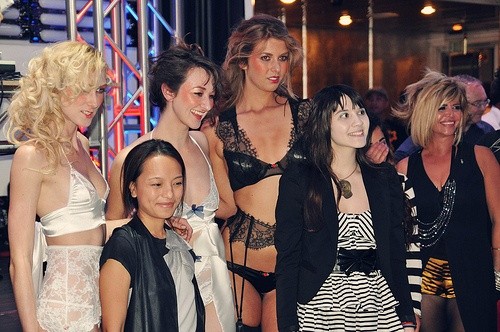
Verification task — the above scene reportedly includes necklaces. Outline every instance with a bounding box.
[337,162,359,199]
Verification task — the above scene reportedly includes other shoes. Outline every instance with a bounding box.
[30,31,41,43]
[17,29,29,40]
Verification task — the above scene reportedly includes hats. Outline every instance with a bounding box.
[363,86,390,99]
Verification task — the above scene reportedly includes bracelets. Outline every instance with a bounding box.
[401,322,416,328]
[493,248,500,291]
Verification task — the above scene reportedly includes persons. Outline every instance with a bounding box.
[363,66,500,165]
[1,40,110,332]
[365,112,422,332]
[273,84,417,332]
[396,72,500,332]
[199,14,314,332]
[99,139,206,332]
[105,35,237,332]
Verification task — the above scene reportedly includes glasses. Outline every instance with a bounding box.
[467,98,490,108]
